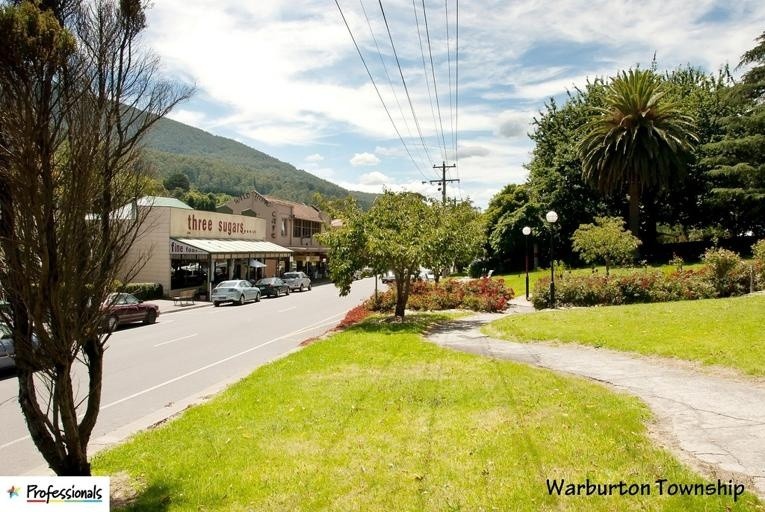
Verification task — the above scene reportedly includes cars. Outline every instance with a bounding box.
[252,276,290,298]
[210,277,262,307]
[0,291,162,380]
[170,263,227,281]
[380,271,395,284]
[424,268,435,280]
[281,271,313,293]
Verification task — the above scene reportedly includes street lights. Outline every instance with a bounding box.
[544,209,560,308]
[521,226,533,301]
